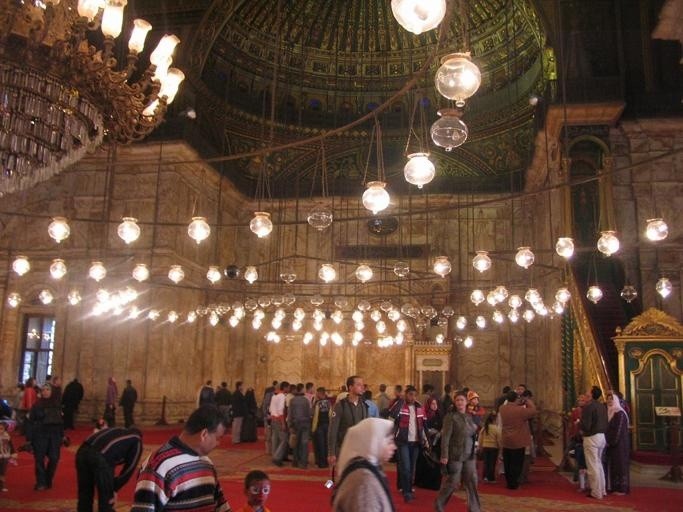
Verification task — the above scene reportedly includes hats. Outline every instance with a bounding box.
[467,391,479,401]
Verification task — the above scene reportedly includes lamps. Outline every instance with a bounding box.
[249,211,273,239]
[207,265,222,284]
[587,286,603,305]
[244,266,258,284]
[187,217,212,244]
[117,216,142,245]
[362,180,390,215]
[306,203,333,232]
[597,231,619,255]
[434,52,482,107]
[391,0,447,35]
[356,262,372,282]
[655,275,672,298]
[473,250,492,273]
[49,259,67,278]
[1,1,191,168]
[280,270,296,283]
[645,218,669,242]
[89,262,107,280]
[619,280,638,302]
[430,109,469,150]
[47,216,71,244]
[168,265,185,283]
[556,237,574,259]
[394,261,410,278]
[12,255,30,275]
[403,154,436,188]
[319,264,336,283]
[0,280,569,346]
[433,256,451,278]
[133,264,148,282]
[515,247,535,269]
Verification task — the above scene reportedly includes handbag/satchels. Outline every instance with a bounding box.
[289,434,297,449]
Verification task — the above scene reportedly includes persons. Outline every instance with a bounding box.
[119,379,137,428]
[93,415,109,434]
[131,405,231,511]
[261,376,543,511]
[1,375,84,492]
[566,385,631,499]
[241,469,271,511]
[75,425,144,511]
[103,376,118,426]
[198,379,258,445]
